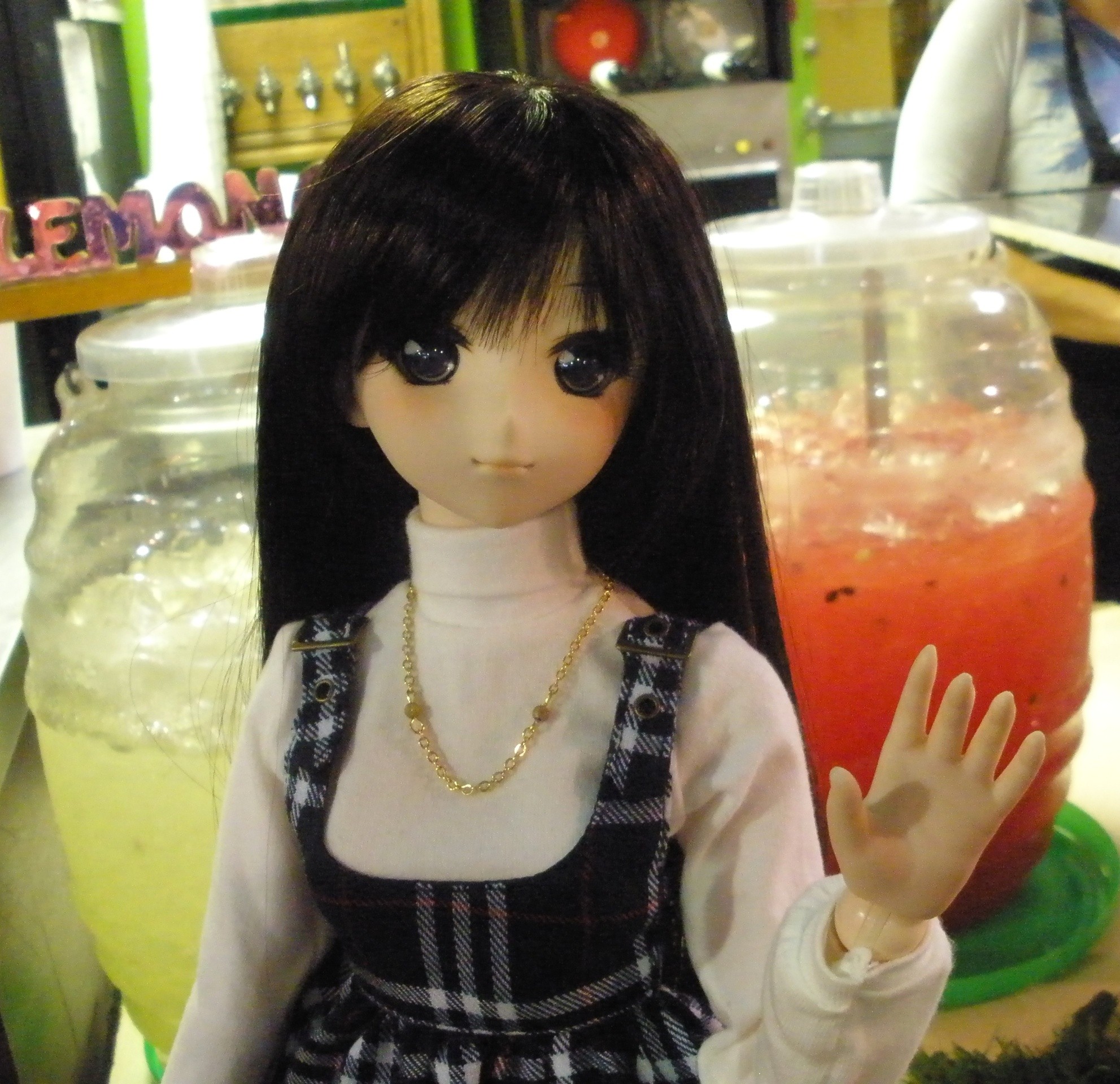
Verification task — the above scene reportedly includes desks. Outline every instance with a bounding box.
[865,187,1120,595]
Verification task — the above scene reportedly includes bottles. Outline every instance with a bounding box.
[548,0,649,94]
[660,0,769,86]
[23,232,265,1063]
[702,159,1096,934]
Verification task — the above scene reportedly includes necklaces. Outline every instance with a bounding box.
[400,564,611,796]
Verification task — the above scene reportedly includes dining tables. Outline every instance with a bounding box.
[108,596,1120,1084]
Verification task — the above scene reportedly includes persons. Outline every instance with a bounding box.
[885,0,1118,602]
[153,68,1052,1084]
